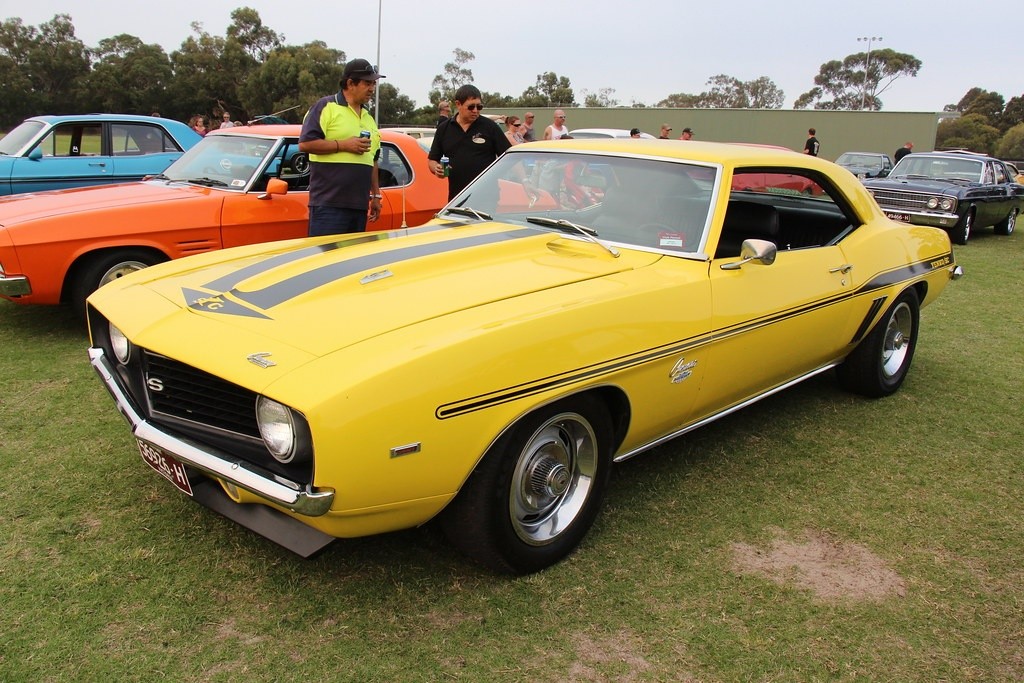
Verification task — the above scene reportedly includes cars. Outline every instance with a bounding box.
[10,125,561,316]
[84,138,951,575]
[0,113,288,203]
[826,147,1024,244]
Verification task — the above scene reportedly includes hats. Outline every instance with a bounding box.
[683,127,696,135]
[342,59,386,81]
[661,123,672,131]
[630,129,640,134]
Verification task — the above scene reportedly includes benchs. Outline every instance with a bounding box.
[663,196,848,248]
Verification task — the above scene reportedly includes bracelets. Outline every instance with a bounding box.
[334,140,339,154]
[372,193,383,200]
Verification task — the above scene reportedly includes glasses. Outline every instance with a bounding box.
[529,115,534,118]
[512,123,521,127]
[554,116,565,118]
[468,104,483,110]
[224,117,229,118]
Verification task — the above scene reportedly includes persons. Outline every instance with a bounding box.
[804,128,820,157]
[152,112,245,137]
[893,141,913,166]
[428,84,593,218]
[299,58,387,237]
[628,123,695,140]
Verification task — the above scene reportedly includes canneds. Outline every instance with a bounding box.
[440,157,450,177]
[360,130,370,149]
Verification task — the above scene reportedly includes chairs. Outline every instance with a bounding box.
[720,201,780,257]
[592,187,661,245]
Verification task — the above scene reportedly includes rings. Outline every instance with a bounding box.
[436,171,438,174]
